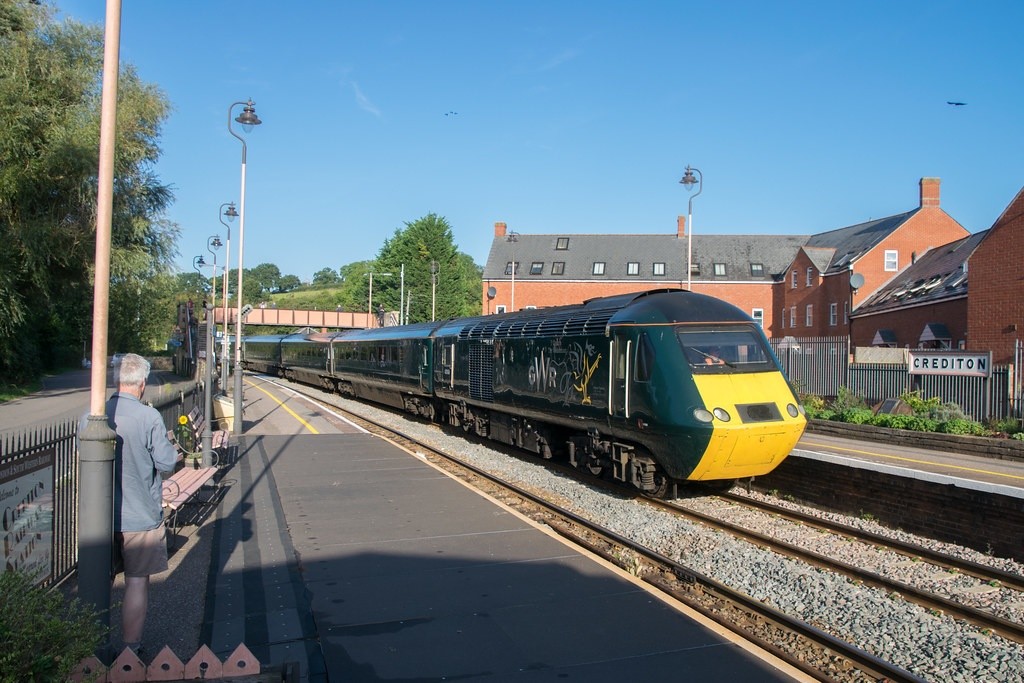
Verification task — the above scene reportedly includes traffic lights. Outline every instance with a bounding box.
[241,304,253,317]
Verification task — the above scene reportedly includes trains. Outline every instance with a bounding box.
[214,288,807,501]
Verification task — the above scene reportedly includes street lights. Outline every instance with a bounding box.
[369,272,393,314]
[220,202,240,396]
[506,230,521,312]
[193,254,206,351]
[228,97,263,435]
[679,164,704,292]
[175,326,182,375]
[208,234,223,356]
[428,258,440,322]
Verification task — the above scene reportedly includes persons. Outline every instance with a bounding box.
[704,346,726,364]
[77,352,178,665]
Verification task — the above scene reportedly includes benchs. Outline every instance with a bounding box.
[153,430,218,551]
[187,406,229,467]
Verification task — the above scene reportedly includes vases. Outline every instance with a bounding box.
[176,456,204,471]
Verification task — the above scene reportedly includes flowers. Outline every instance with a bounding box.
[177,416,191,444]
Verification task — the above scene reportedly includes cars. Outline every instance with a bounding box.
[110,354,124,368]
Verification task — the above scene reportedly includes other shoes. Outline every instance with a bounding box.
[122,643,147,662]
